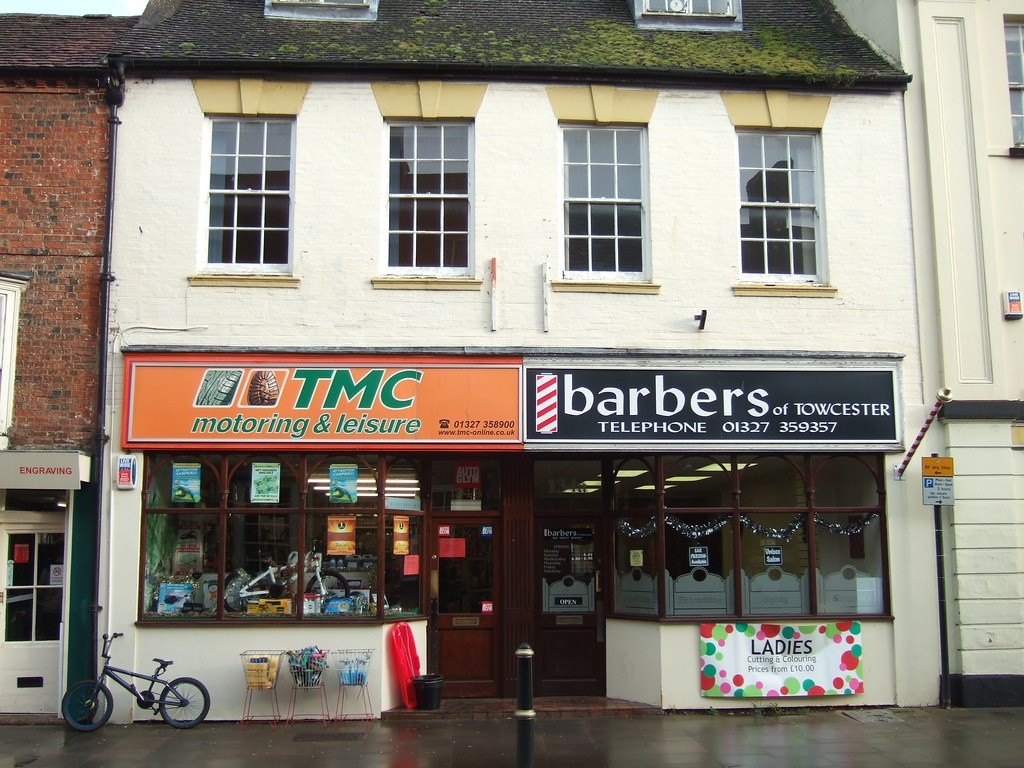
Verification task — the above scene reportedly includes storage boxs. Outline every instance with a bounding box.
[259,599,295,615]
[157,583,194,615]
[370,594,390,610]
[349,589,370,611]
[326,589,345,599]
[247,604,258,614]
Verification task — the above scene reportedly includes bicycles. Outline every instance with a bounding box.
[61,633,212,732]
[224,538,351,613]
